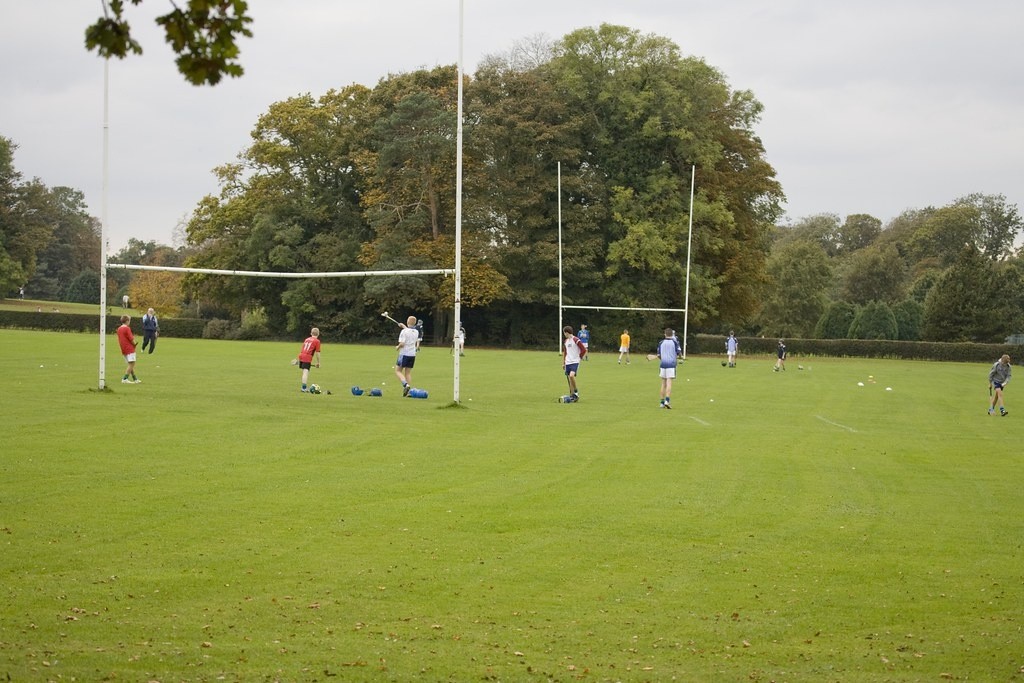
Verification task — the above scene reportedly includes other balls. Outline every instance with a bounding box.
[384,312,389,315]
[469,398,473,402]
[382,382,386,386]
[687,378,690,382]
[710,399,714,402]
[392,367,395,369]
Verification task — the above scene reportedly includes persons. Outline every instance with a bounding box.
[725,331,738,368]
[452,322,467,356]
[986,355,1012,417]
[617,329,631,365]
[52,308,59,313]
[122,294,129,309]
[773,338,787,373]
[117,315,142,384]
[141,307,158,355]
[298,328,321,392]
[657,328,682,410]
[395,315,424,397]
[37,306,42,313]
[562,324,589,402]
[18,284,25,300]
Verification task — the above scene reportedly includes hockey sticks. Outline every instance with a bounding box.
[290,359,321,369]
[989,387,998,417]
[381,311,404,328]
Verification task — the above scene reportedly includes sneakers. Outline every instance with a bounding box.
[134,380,142,383]
[122,379,133,384]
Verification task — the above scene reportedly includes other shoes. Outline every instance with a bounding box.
[988,409,993,414]
[402,384,410,397]
[660,404,664,408]
[574,392,579,401]
[664,401,671,408]
[301,388,309,392]
[1001,410,1008,416]
[141,348,144,354]
